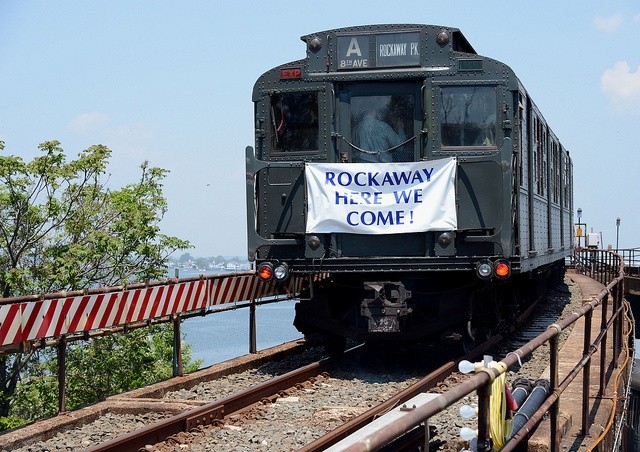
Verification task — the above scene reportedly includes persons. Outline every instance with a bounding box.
[352,101,406,164]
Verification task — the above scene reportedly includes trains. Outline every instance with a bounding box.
[243,23,575,375]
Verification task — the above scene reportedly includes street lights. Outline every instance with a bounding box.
[576,208,583,276]
[616,217,621,253]
